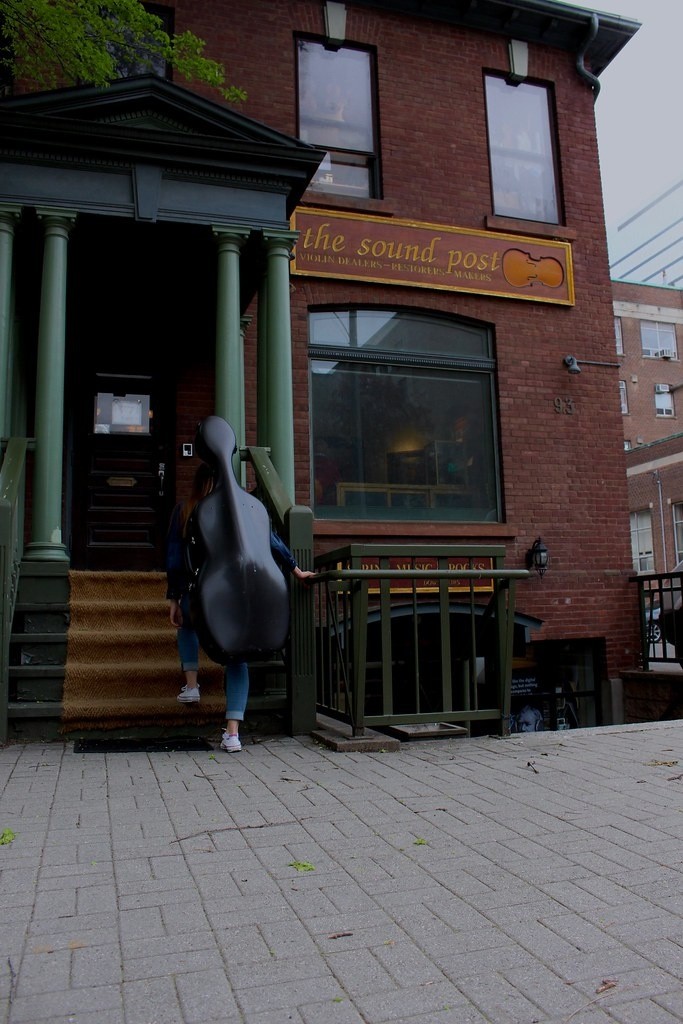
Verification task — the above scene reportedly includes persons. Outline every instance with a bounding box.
[167,463,315,753]
[515,704,543,734]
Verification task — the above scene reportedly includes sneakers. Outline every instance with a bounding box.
[220,726,242,753]
[177,683,202,703]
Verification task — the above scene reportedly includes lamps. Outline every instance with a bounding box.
[525,535,549,579]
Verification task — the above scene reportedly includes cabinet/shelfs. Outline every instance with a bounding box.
[335,440,482,508]
